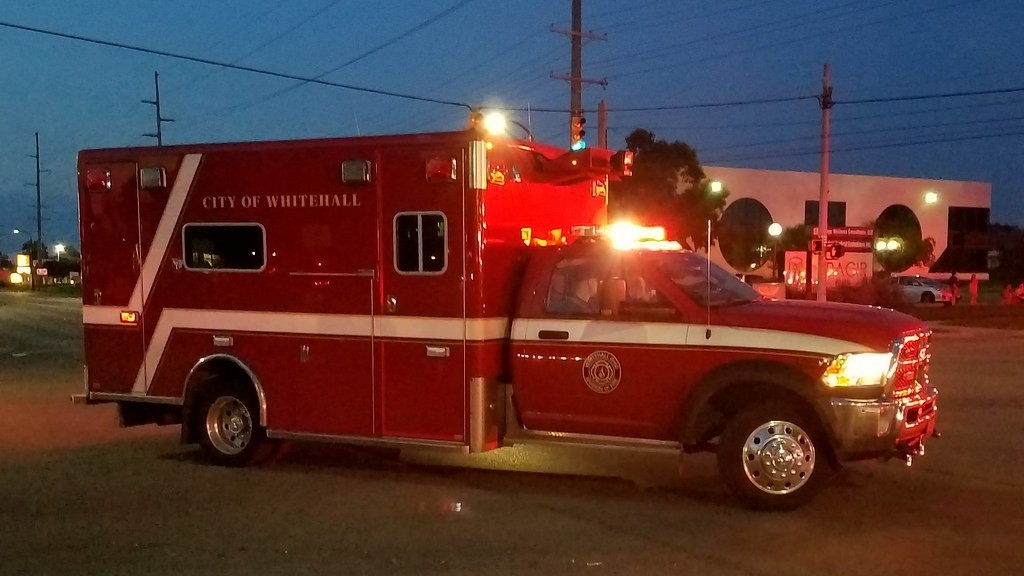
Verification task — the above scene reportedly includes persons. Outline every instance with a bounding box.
[949,270,959,306]
[70,279,74,285]
[546,260,601,315]
[970,274,979,305]
[1001,283,1024,305]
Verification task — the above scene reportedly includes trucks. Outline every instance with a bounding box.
[68,131,939,508]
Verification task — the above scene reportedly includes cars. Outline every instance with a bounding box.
[889,274,953,306]
[734,273,764,287]
[70,276,80,285]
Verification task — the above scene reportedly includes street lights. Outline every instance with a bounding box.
[13,230,32,259]
[877,239,898,271]
[56,243,65,261]
[767,222,781,281]
